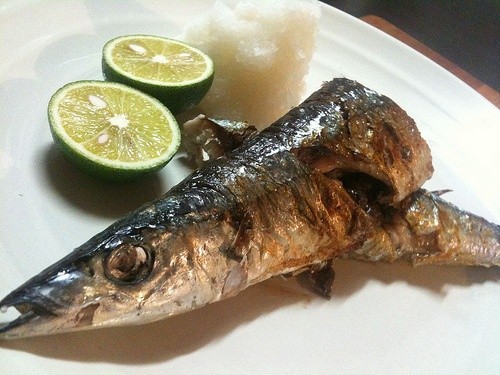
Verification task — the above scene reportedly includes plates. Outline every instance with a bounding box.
[0,0,499,374]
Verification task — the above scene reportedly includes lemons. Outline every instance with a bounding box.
[48,34,216,183]
